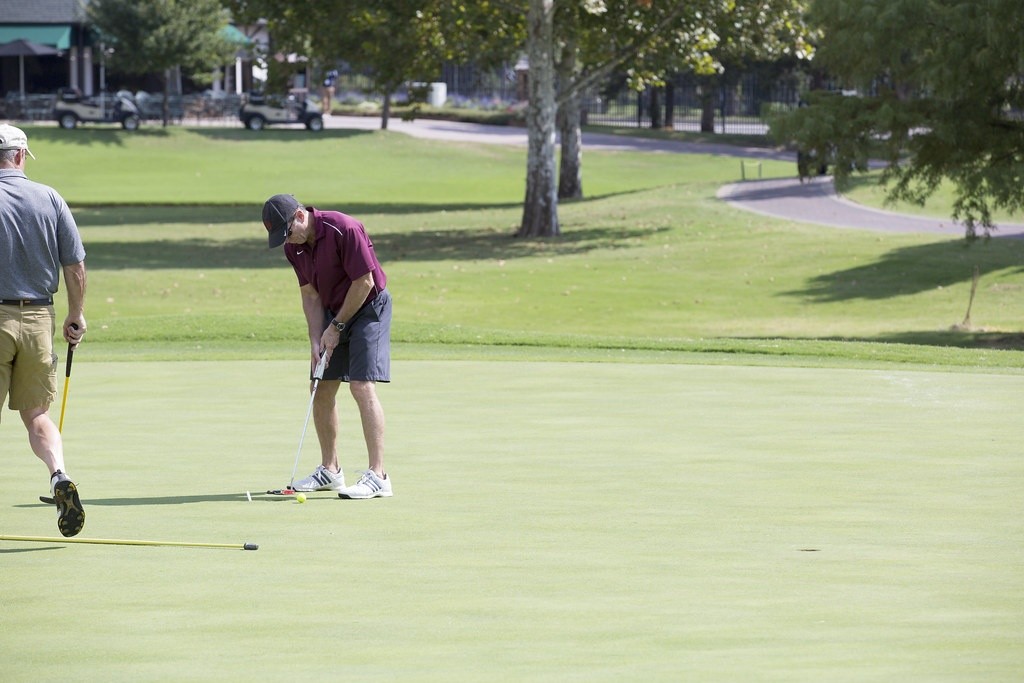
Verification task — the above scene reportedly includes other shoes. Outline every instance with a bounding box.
[52,474,85,538]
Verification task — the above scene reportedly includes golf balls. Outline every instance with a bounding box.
[297,494,307,502]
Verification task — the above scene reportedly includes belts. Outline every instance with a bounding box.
[0,298,53,305]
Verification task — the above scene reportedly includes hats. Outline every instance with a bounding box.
[0,124,36,160]
[262,194,298,249]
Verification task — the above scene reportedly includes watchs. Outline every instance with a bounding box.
[332,319,345,331]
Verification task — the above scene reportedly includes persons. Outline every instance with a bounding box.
[0,124,86,536]
[117,86,149,125]
[263,195,393,499]
[321,70,336,120]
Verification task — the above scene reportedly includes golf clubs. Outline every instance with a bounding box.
[3,534,261,551]
[266,348,327,496]
[39,322,80,504]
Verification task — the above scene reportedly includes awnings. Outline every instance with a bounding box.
[0,26,71,49]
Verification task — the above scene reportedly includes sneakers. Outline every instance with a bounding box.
[338,465,394,499]
[287,464,345,491]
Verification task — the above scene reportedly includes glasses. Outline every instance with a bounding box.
[288,213,296,236]
